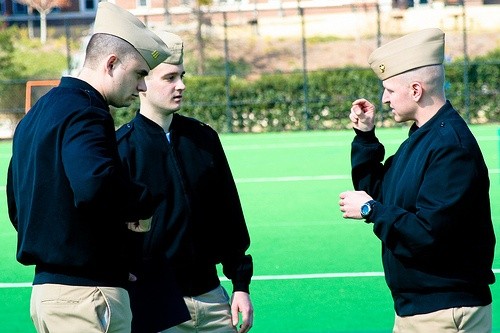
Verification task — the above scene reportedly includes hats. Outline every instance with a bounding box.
[150,25,184,65]
[368,28,446,81]
[93,1,172,70]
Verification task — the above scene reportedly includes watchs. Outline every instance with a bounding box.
[360,200,377,219]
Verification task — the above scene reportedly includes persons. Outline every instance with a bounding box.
[115,31,253,333]
[7,3,171,332]
[339,28,496,333]
